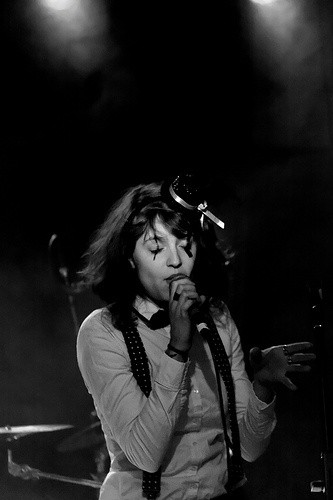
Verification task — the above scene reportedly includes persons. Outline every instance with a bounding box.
[73,173,316,500]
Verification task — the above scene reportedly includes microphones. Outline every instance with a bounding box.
[170,274,211,341]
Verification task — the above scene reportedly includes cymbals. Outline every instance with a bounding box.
[0,423,75,444]
[54,421,105,453]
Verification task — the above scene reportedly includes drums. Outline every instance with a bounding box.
[228,448,248,492]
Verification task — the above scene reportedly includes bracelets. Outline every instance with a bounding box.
[166,343,189,359]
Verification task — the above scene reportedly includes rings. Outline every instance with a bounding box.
[282,344,290,355]
[287,354,293,365]
